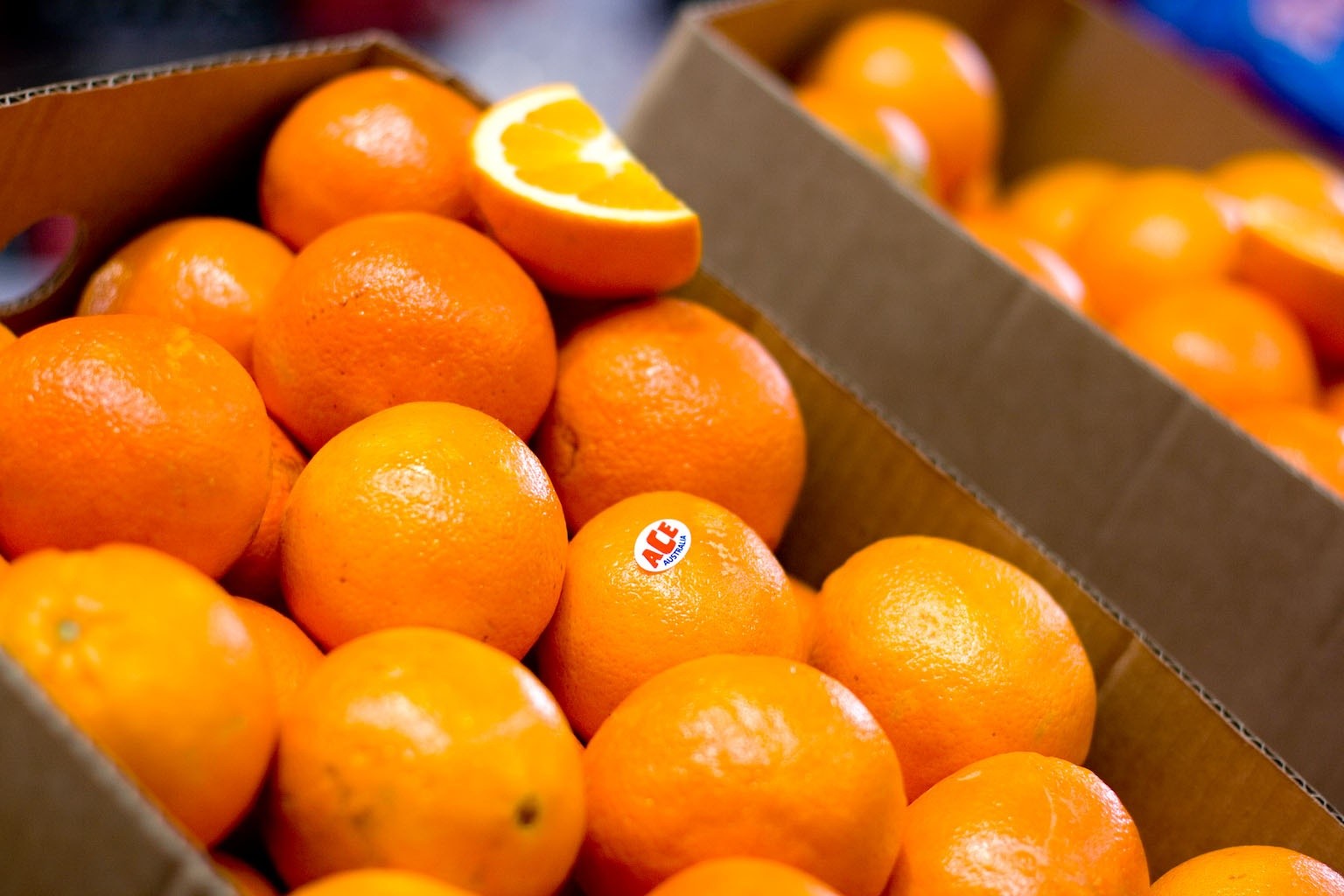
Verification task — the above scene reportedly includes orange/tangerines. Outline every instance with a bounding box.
[773,11,1344,519]
[0,63,1344,896]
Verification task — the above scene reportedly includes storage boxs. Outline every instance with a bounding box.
[1,2,1342,892]
